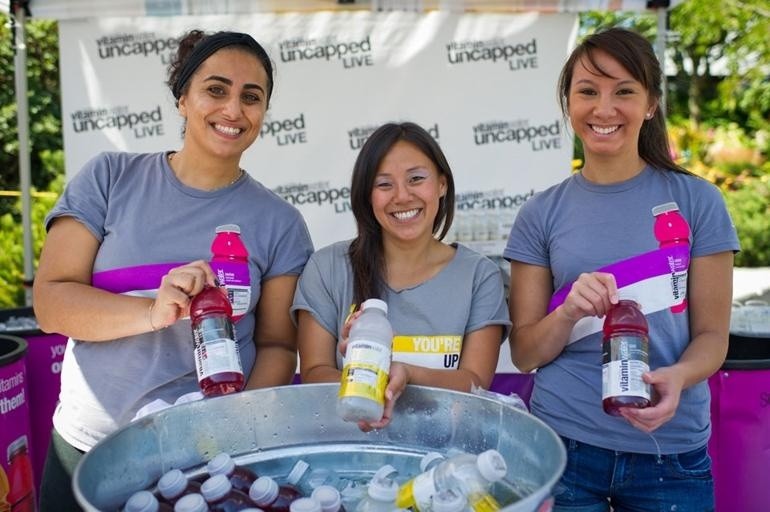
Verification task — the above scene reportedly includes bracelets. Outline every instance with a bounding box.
[149,300,168,333]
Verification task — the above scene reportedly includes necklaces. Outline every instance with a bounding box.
[168,152,243,185]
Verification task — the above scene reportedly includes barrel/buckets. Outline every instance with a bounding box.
[72,381,568,512]
[0,336,39,511]
[0,307,69,486]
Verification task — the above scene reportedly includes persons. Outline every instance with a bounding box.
[31,28,313,511]
[501,25,742,512]
[289,120,515,432]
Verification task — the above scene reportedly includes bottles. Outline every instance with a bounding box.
[188,272,250,398]
[120,448,511,511]
[209,223,255,322]
[8,433,37,511]
[652,200,695,313]
[336,292,398,427]
[601,290,654,417]
